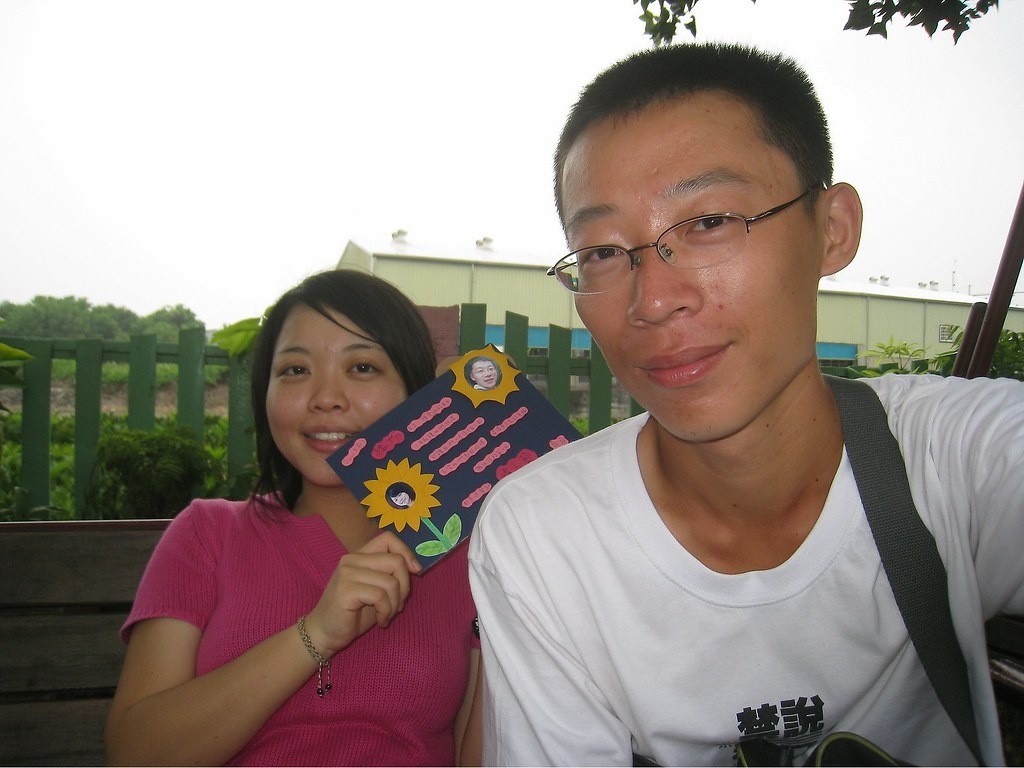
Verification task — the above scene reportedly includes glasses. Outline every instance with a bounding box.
[545,180,829,295]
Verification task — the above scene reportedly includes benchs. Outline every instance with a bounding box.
[0,531,170,768]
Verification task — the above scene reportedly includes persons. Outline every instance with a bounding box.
[101,267,486,768]
[466,42,1024,767]
[386,481,417,512]
[469,357,499,391]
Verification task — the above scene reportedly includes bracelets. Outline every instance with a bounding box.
[296,616,332,697]
